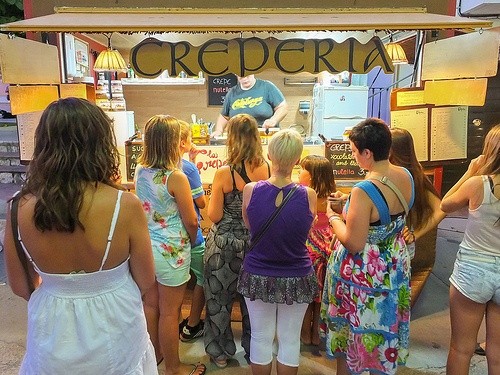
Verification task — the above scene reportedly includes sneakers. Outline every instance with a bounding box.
[180,319,206,342]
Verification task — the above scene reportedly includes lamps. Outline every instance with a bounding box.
[93,33,128,72]
[385,31,409,65]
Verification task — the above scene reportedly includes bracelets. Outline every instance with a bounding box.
[411,233,416,243]
[328,215,341,223]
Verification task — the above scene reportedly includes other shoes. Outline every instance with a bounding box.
[474,342,487,356]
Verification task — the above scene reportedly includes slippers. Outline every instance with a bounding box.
[178,361,207,375]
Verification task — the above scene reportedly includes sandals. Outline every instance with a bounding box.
[210,354,228,368]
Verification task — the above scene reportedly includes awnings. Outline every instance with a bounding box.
[0,6,493,33]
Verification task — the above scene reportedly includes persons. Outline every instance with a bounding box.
[133,114,206,375]
[202,113,273,369]
[4,97,160,375]
[388,128,448,261]
[210,73,288,138]
[178,120,206,342]
[319,117,415,375]
[441,122,500,375]
[297,155,337,347]
[235,128,319,375]
